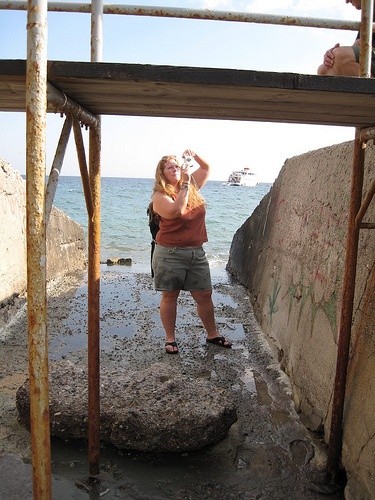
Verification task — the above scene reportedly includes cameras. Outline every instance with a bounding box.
[185,156,194,167]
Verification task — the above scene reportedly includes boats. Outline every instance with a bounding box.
[227,167,259,191]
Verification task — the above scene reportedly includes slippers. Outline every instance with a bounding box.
[165,341,178,354]
[206,335,232,348]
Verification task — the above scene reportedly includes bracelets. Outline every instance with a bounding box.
[181,181,188,191]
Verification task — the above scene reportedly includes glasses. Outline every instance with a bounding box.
[162,155,177,160]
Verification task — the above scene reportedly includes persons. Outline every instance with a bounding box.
[150,149,232,354]
[317,0,375,76]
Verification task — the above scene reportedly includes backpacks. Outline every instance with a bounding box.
[147,202,160,278]
